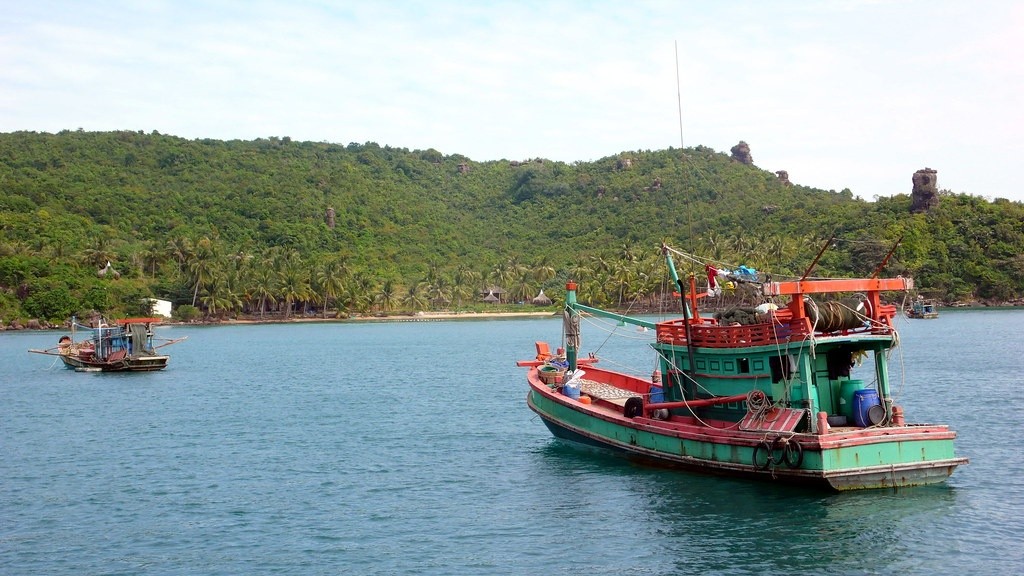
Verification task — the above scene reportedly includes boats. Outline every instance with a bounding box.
[515,232,970,493]
[905,292,940,319]
[28,313,188,372]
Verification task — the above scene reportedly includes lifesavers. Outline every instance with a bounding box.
[623,396,644,419]
[752,435,806,473]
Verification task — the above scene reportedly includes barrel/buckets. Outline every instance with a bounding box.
[853,389,880,427]
[542,367,559,372]
[538,365,565,384]
[840,380,864,423]
